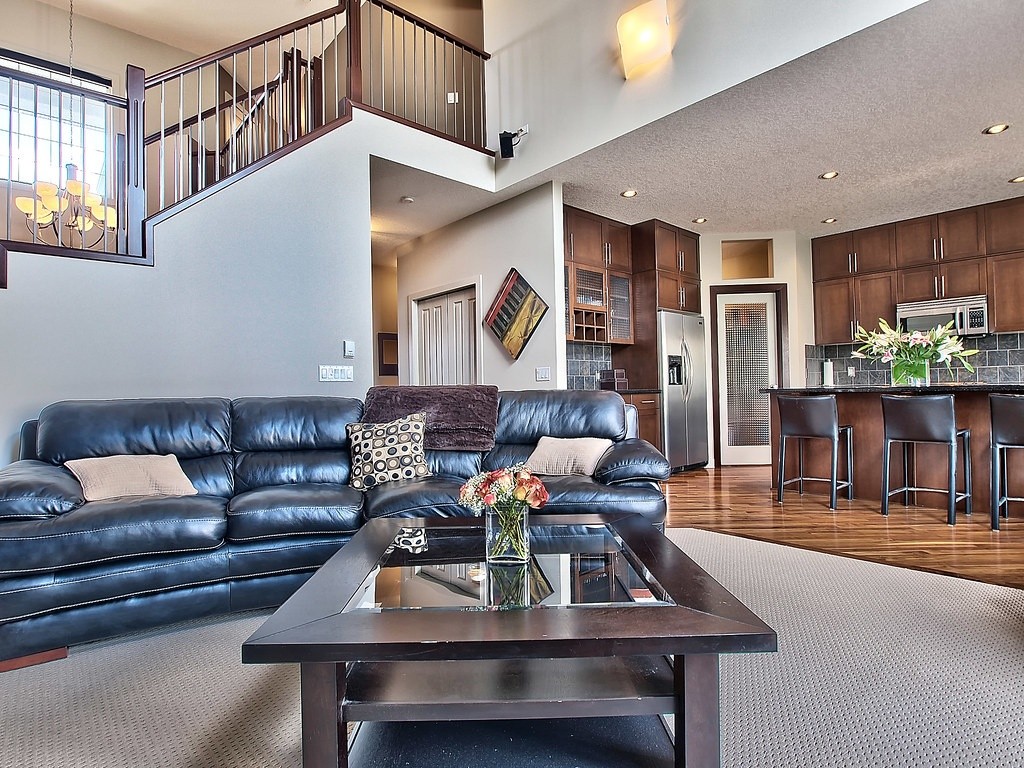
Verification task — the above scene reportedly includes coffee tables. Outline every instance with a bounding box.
[241,513,779,767]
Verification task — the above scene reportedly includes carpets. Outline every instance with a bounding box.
[0,526,1023,768]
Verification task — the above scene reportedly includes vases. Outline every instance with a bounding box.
[486,560,531,607]
[891,359,930,387]
[486,501,531,559]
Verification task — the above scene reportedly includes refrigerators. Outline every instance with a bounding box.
[657,309,707,472]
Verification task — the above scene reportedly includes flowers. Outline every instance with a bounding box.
[849,318,979,383]
[458,462,549,559]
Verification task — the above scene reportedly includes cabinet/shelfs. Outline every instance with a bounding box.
[618,394,661,454]
[811,195,1024,347]
[563,204,701,345]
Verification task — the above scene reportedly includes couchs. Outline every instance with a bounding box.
[0,389,671,673]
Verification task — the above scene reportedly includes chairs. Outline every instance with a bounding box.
[777,394,854,511]
[879,394,972,524]
[989,394,1023,532]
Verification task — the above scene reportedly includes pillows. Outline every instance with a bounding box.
[64,453,198,501]
[526,435,615,476]
[345,411,433,491]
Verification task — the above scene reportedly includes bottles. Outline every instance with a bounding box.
[824,359,834,385]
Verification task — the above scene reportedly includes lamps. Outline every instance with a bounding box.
[15,0,119,250]
[616,0,671,83]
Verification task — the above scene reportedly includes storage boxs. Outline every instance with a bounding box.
[599,369,628,391]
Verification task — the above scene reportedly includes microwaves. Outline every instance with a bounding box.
[895,295,988,339]
